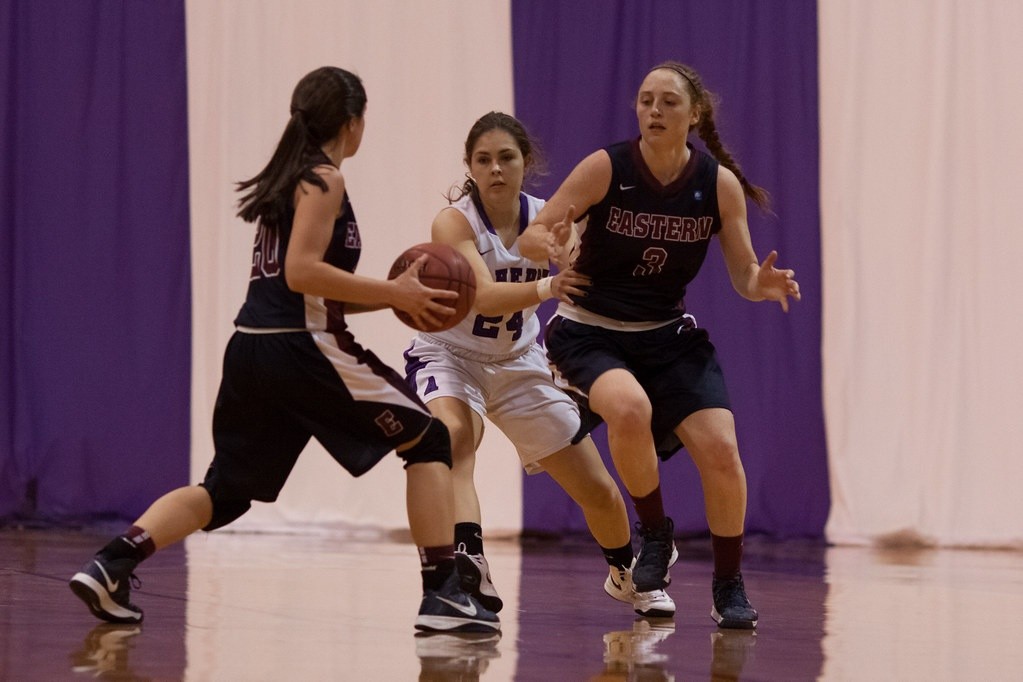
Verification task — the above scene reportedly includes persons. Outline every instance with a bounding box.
[402,111,676,617]
[517,61,801,629]
[68,66,500,633]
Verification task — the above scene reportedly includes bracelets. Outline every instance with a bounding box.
[536,276,554,302]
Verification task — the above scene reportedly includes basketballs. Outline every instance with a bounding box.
[387,242,477,333]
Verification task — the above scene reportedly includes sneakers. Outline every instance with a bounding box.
[454,543,503,614]
[414,574,501,633]
[711,574,759,630]
[632,518,679,593]
[69,554,144,623]
[603,557,677,617]
